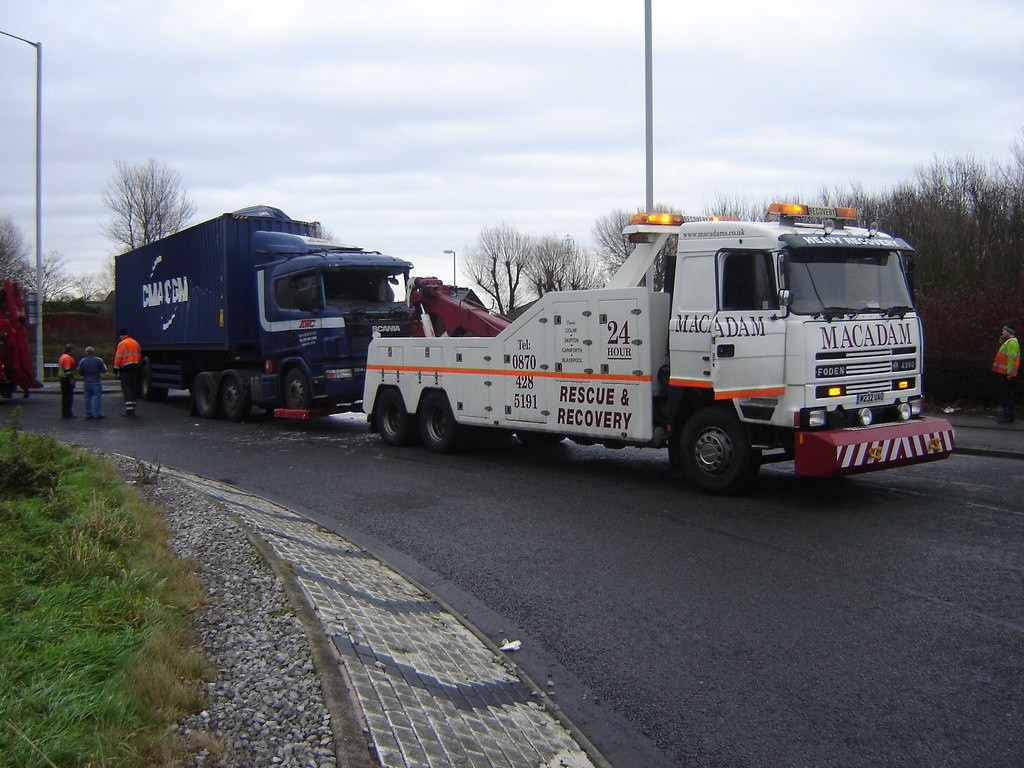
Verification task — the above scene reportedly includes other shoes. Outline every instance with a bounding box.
[121,412,133,417]
[94,415,105,419]
[87,415,96,419]
[68,415,77,419]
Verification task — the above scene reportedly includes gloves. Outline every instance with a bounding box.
[69,379,77,390]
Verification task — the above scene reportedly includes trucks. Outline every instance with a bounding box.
[0,277,45,398]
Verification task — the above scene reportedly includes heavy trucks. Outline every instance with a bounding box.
[115,203,419,423]
[361,203,957,499]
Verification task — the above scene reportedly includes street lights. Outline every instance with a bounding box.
[444,250,456,288]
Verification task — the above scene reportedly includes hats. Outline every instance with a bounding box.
[119,328,128,335]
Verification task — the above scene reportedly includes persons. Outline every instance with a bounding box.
[76,345,108,419]
[992,324,1020,424]
[114,329,141,419]
[58,344,77,419]
